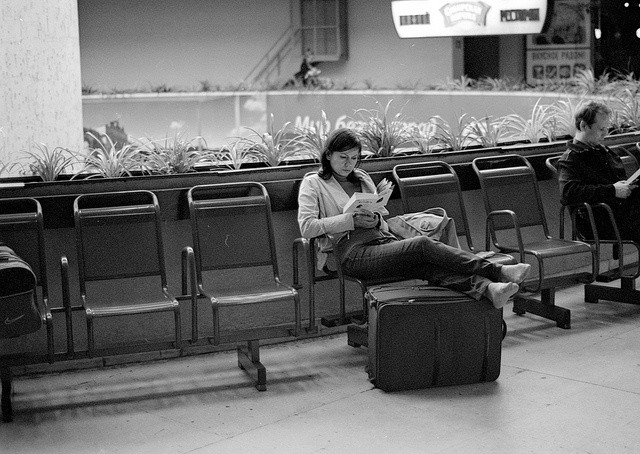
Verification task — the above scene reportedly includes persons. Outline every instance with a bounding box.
[296,125,532,311]
[553,100,640,249]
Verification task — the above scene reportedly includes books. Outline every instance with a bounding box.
[342,177,395,216]
[623,164,640,189]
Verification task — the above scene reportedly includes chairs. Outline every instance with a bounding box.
[72,189,183,355]
[392,161,518,279]
[0,197,55,424]
[186,180,301,394]
[542,151,640,303]
[472,152,601,329]
[292,176,405,326]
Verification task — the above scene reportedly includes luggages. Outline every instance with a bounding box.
[363,283,507,392]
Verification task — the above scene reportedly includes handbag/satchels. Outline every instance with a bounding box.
[0,242,43,339]
[386,207,462,251]
